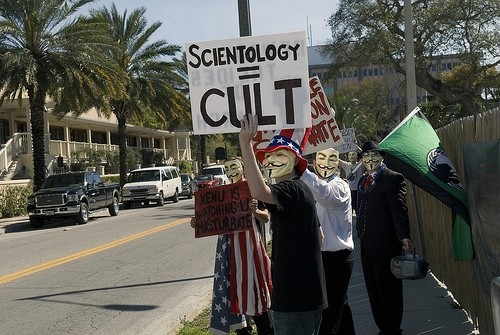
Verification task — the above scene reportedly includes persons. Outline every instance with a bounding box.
[355,140,412,335]
[339,150,366,238]
[238,113,329,335]
[299,148,356,335]
[190,156,275,335]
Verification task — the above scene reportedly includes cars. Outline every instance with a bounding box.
[193,174,220,191]
[179,174,198,200]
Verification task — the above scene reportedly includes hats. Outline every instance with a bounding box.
[255,135,308,178]
[357,140,385,159]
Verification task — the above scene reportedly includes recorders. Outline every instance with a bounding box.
[391,247,428,280]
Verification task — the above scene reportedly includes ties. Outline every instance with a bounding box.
[364,175,373,190]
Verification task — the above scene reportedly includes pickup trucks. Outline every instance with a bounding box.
[26,170,122,226]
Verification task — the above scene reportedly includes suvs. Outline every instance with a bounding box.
[202,164,231,185]
[121,166,182,208]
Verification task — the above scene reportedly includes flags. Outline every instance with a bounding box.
[377,108,474,262]
